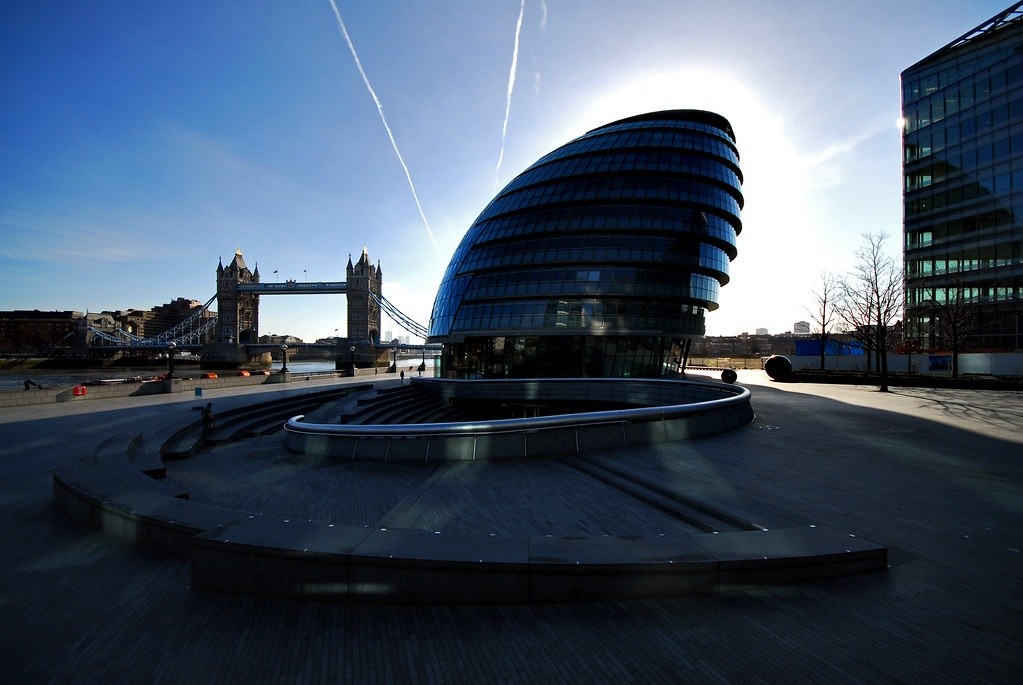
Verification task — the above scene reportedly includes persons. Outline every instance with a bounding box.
[201,402,215,434]
[400,370,405,384]
